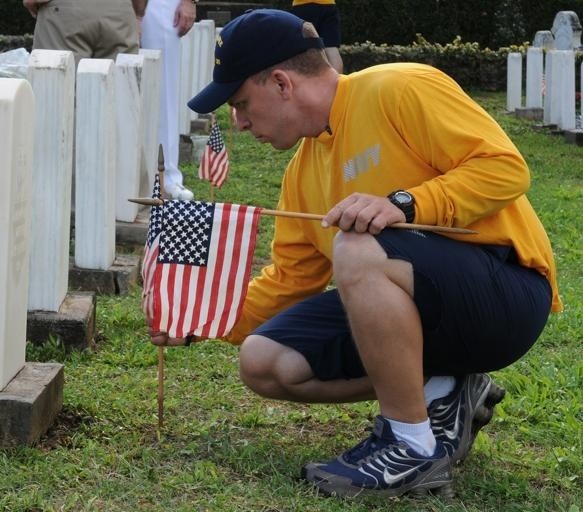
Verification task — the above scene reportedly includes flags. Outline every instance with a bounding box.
[151,196,261,341]
[199,122,231,187]
[140,173,162,320]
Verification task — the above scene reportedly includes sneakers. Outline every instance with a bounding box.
[301,373,504,498]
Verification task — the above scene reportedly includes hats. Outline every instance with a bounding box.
[187,8,325,114]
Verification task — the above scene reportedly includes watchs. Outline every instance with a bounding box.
[384,189,415,224]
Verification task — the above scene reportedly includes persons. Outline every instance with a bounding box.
[22,0,147,60]
[185,7,562,501]
[291,0,345,68]
[140,1,200,202]
